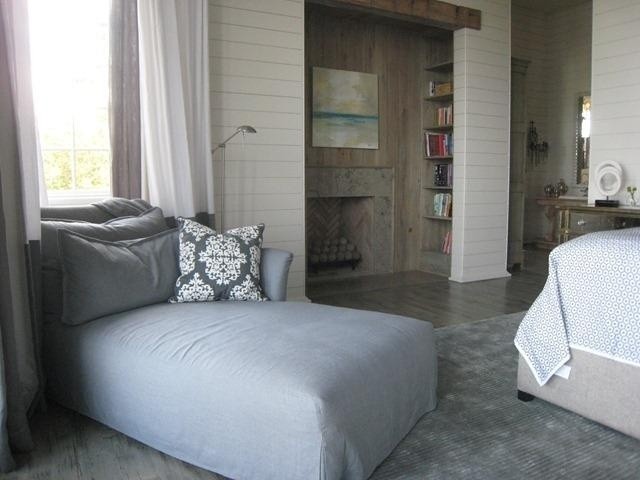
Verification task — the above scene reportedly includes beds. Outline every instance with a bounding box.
[516,224,639,441]
[32,198,437,475]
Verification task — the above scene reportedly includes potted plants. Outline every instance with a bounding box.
[627,186,639,207]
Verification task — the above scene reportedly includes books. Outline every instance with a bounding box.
[424,103,453,256]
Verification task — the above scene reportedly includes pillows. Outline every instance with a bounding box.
[36,207,271,327]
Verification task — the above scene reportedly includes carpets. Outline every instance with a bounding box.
[0,306,639,475]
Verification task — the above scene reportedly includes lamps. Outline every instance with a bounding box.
[210,124,256,162]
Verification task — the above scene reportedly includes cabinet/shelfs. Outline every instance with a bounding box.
[422,32,454,277]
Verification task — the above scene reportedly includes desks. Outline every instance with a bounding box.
[557,205,640,247]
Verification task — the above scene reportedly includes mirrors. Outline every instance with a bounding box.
[576,92,589,187]
